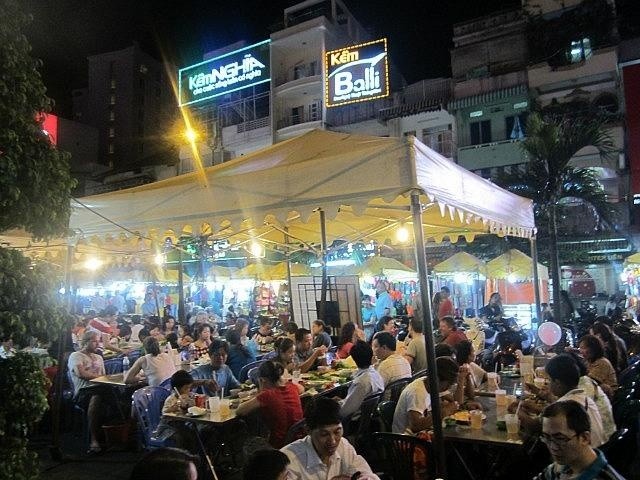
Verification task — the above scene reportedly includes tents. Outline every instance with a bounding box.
[150,268,191,285]
[52,126,548,480]
[230,263,266,278]
[260,260,309,281]
[360,256,419,285]
[205,266,235,278]
[433,252,488,302]
[623,251,640,268]
[487,247,549,305]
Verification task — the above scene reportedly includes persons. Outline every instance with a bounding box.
[390,357,467,480]
[518,353,607,451]
[482,331,524,371]
[335,321,367,358]
[178,324,195,350]
[593,316,626,353]
[311,319,333,349]
[255,337,296,376]
[67,331,112,454]
[372,332,412,400]
[22,336,47,353]
[439,317,471,353]
[566,348,618,440]
[234,317,249,346]
[549,302,554,317]
[532,400,627,480]
[226,330,256,381]
[389,283,403,316]
[117,337,176,444]
[0,338,14,359]
[284,322,298,338]
[603,296,621,318]
[333,342,385,419]
[86,306,131,356]
[191,313,217,335]
[457,339,488,388]
[251,321,276,344]
[376,281,393,321]
[375,316,397,336]
[588,323,628,374]
[156,369,199,480]
[577,336,618,397]
[189,340,244,395]
[437,286,453,325]
[227,305,237,321]
[282,396,382,479]
[141,292,155,317]
[479,293,506,331]
[138,326,150,343]
[542,302,549,318]
[188,323,212,361]
[560,290,574,315]
[131,315,144,340]
[163,316,175,337]
[167,333,179,360]
[434,342,465,393]
[294,328,327,373]
[244,446,292,480]
[404,318,427,368]
[149,323,160,337]
[236,359,305,449]
[361,295,376,342]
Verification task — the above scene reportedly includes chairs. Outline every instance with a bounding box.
[377,396,399,432]
[286,419,310,443]
[131,385,181,445]
[387,371,415,397]
[234,359,267,379]
[263,350,278,359]
[412,368,428,382]
[369,432,438,480]
[614,353,640,449]
[103,358,127,374]
[119,349,142,360]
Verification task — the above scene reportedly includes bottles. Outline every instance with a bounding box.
[123,357,130,377]
[189,343,195,361]
[195,394,205,407]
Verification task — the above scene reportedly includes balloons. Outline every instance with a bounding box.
[537,322,562,350]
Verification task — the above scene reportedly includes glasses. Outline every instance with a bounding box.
[538,432,579,445]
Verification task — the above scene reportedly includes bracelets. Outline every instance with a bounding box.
[466,371,472,378]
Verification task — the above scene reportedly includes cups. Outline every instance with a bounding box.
[495,389,505,404]
[219,400,231,416]
[470,409,481,430]
[487,372,498,388]
[504,414,519,434]
[293,371,300,381]
[534,378,545,388]
[205,397,218,413]
